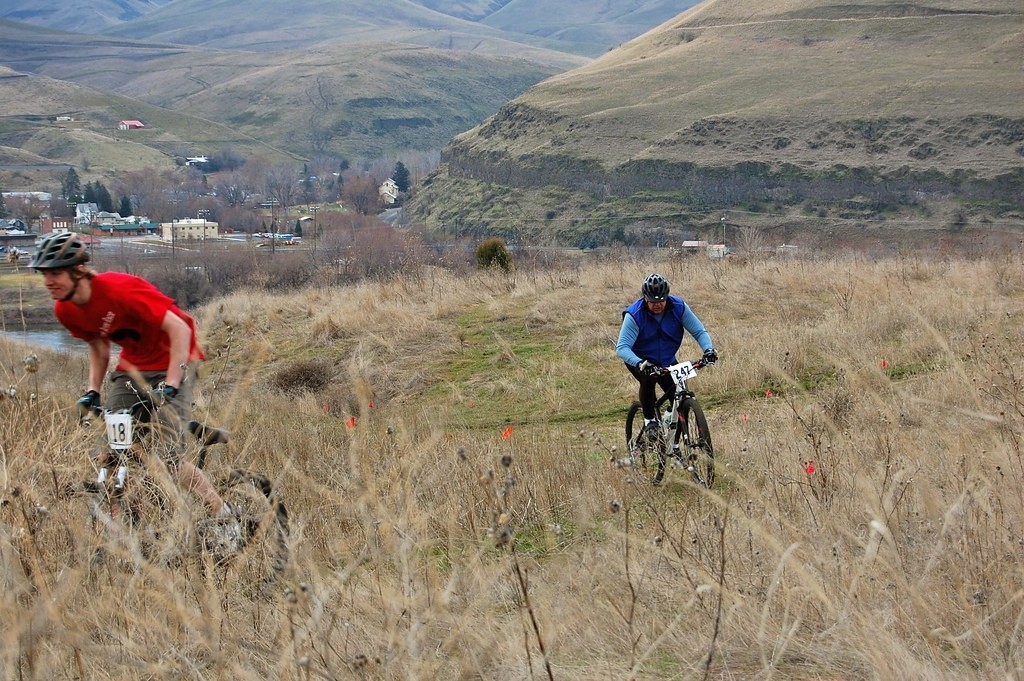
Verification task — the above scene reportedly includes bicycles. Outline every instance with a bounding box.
[625,352,719,490]
[20,390,293,612]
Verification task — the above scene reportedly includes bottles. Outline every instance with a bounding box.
[662,406,673,428]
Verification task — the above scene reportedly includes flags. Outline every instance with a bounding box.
[767,389,772,397]
[369,400,373,407]
[807,463,815,475]
[882,361,886,368]
[346,417,354,429]
[503,426,511,440]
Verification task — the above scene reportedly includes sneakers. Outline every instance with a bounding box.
[644,422,661,441]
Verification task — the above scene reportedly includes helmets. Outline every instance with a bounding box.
[642,274,670,303]
[28,232,90,268]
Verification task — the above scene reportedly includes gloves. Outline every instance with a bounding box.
[77,391,100,417]
[640,361,661,378]
[147,386,174,406]
[702,348,715,365]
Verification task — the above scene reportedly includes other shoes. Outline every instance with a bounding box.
[214,522,241,556]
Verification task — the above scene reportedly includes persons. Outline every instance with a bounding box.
[27,232,241,561]
[615,273,717,439]
